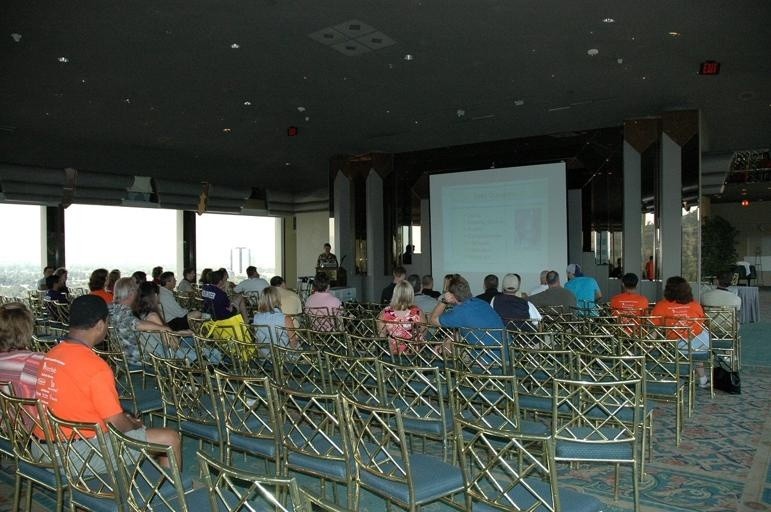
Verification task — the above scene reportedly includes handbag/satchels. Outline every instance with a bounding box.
[200,313,256,362]
[713,367,740,394]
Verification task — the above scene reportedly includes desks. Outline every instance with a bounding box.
[737,285,760,324]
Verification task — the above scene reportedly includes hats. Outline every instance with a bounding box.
[566,263,582,274]
[502,273,521,292]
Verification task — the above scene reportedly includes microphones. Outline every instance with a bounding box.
[340,254,347,266]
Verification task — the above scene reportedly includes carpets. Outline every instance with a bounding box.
[0,360,771,511]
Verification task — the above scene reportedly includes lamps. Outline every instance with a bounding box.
[735,183,753,210]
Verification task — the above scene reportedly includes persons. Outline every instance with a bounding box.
[610,256,742,389]
[37,267,73,304]
[377,265,602,368]
[403,245,415,264]
[29,296,184,478]
[87,266,347,407]
[317,243,338,267]
[0,298,46,456]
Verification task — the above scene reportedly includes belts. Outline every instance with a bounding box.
[32,433,89,444]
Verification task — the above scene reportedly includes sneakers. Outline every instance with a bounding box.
[698,381,710,388]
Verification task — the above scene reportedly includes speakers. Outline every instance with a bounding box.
[328,287,356,300]
